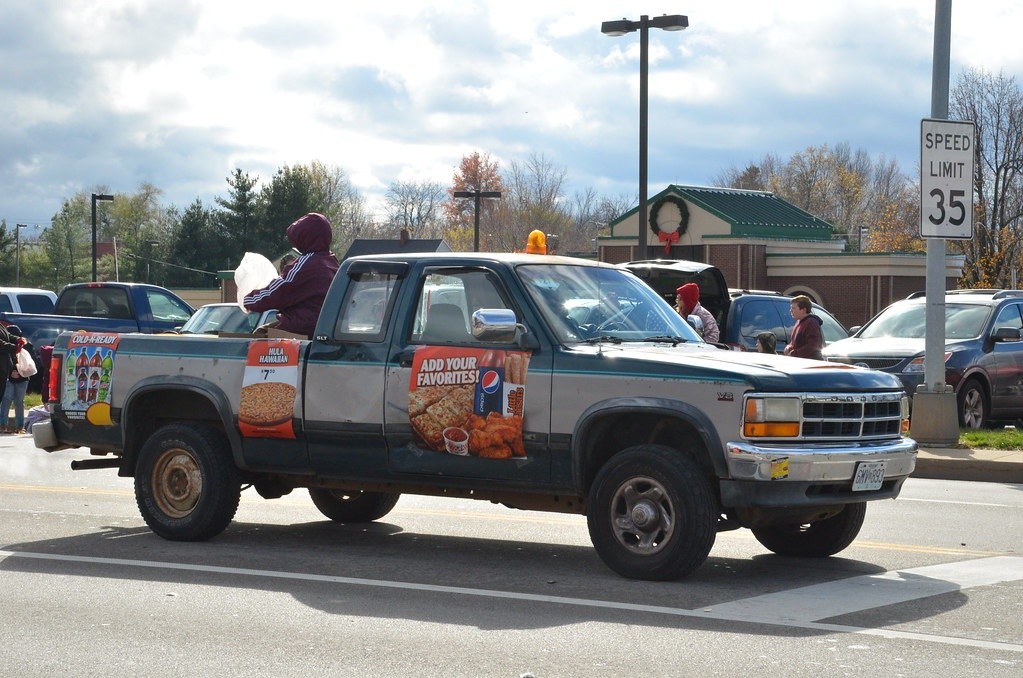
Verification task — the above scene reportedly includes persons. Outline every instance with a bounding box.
[784,295,826,361]
[245,212,340,339]
[673,283,719,343]
[756,332,778,355]
[0,320,53,434]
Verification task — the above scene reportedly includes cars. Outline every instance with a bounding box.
[616,259,852,360]
[818,290,1023,433]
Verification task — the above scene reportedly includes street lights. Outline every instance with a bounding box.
[90,192,114,283]
[454,188,501,252]
[16,222,27,286]
[599,15,689,264]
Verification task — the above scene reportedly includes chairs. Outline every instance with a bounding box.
[420,303,469,341]
[75,301,92,314]
[108,304,127,315]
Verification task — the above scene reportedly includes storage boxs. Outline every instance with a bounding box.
[218,328,308,341]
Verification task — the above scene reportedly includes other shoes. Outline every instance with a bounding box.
[17,428,26,434]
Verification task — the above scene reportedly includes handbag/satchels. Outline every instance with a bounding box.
[16,348,38,377]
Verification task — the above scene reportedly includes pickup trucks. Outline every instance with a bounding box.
[35,252,917,583]
[0,282,196,400]
